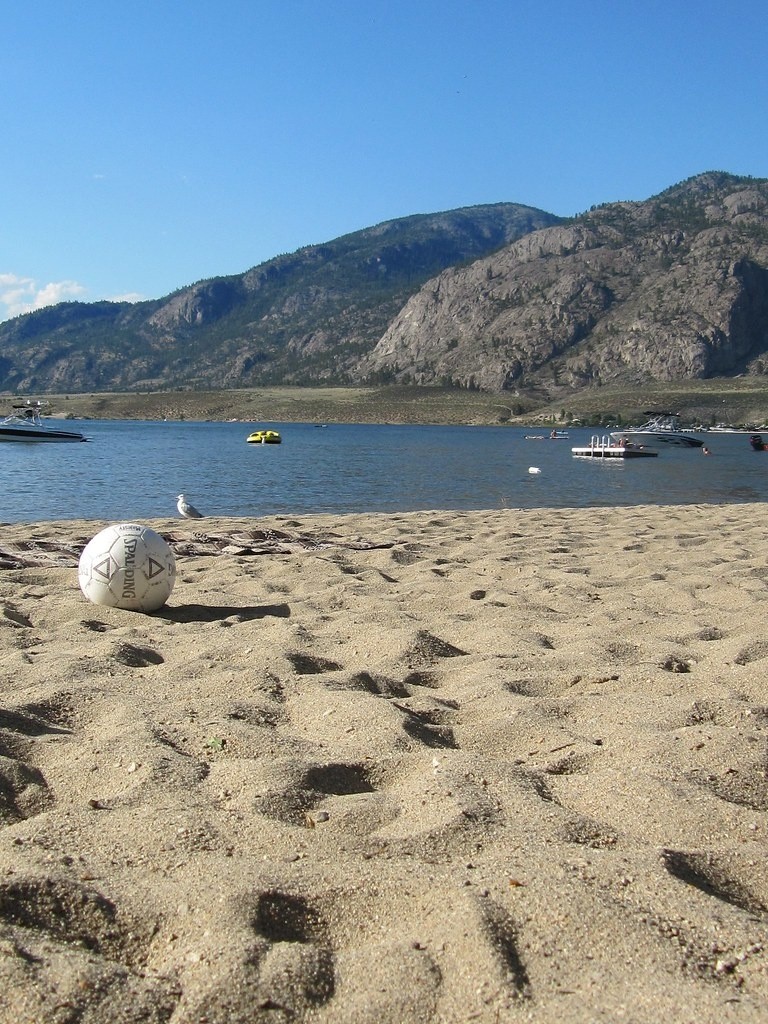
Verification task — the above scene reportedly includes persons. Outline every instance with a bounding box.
[551,429,558,437]
[618,439,624,448]
[703,447,711,455]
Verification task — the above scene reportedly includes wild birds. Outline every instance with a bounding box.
[174,494,205,519]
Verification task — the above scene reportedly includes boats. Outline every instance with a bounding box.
[247,429,282,445]
[609,410,768,451]
[571,435,658,457]
[0,407,94,442]
[523,430,569,440]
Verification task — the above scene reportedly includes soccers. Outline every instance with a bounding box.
[77,521,178,615]
